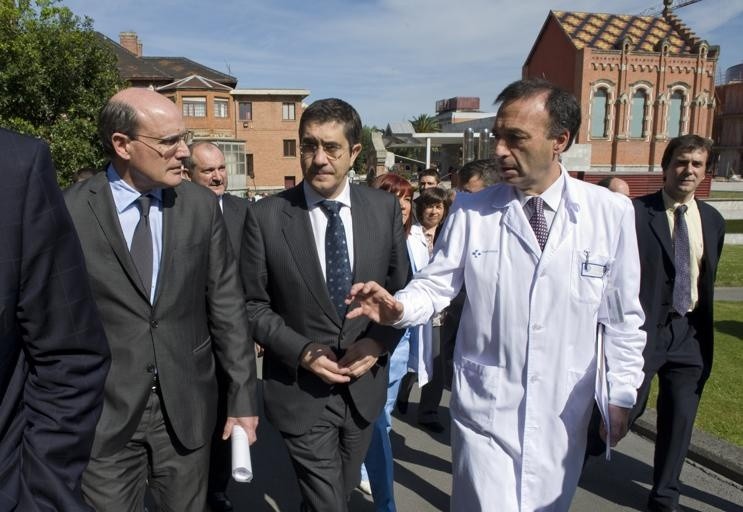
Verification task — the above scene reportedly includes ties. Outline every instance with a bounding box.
[672,206,691,318]
[317,200,353,321]
[130,197,153,299]
[526,197,548,252]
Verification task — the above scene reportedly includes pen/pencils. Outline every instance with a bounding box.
[584,250,590,270]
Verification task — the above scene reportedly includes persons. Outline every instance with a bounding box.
[2,79,648,512]
[588,134,727,512]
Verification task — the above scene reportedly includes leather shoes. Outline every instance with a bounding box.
[647,495,683,512]
[418,422,445,433]
[396,399,408,415]
[359,479,372,495]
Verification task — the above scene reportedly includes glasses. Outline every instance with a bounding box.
[134,131,194,151]
[298,143,349,155]
